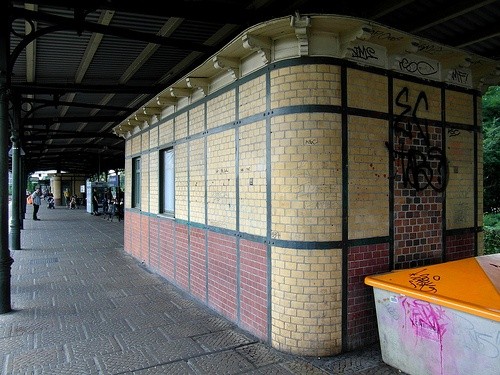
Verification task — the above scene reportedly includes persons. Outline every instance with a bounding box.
[64,188,72,208]
[93,188,99,215]
[31,188,41,221]
[69,194,80,209]
[48,191,53,197]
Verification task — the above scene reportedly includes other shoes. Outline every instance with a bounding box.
[93,211,98,214]
[37,219,40,220]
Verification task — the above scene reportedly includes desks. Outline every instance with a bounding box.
[364,252,500,375]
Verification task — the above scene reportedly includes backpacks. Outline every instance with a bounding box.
[26,195,32,204]
